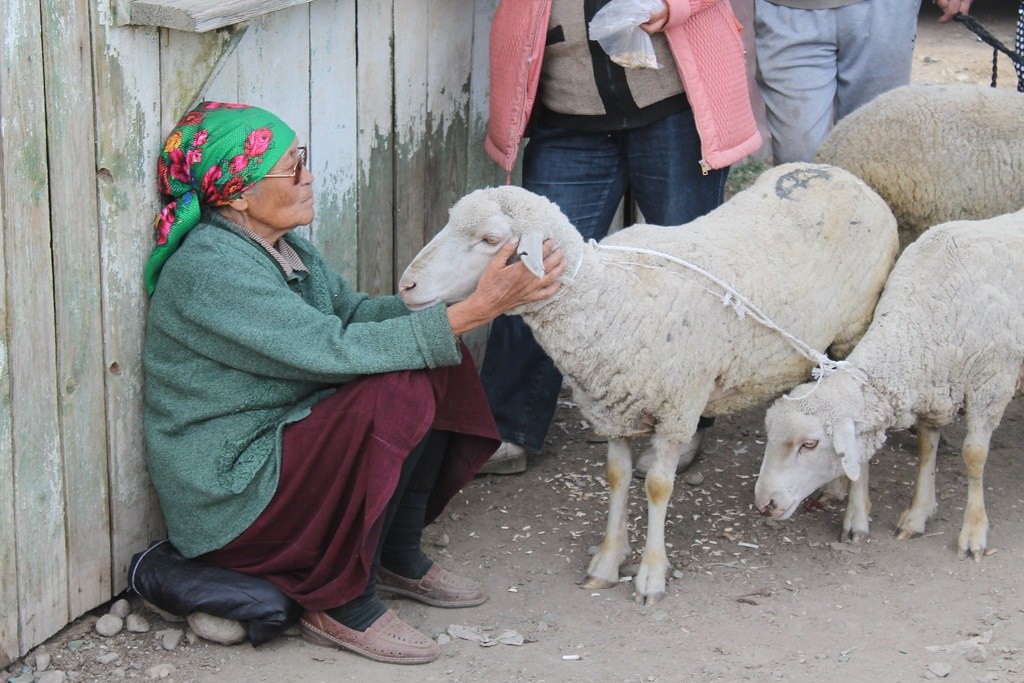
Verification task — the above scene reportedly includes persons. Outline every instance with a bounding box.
[474,0,763,477]
[753,0,972,166]
[142,100,567,667]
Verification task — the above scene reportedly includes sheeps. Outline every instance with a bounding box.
[395,159,901,608]
[727,82,1024,247]
[754,208,1024,561]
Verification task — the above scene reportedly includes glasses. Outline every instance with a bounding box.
[262,144,307,186]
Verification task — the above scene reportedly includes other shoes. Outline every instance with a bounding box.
[476,442,527,474]
[635,428,703,478]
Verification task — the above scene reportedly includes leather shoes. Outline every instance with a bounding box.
[299,608,440,665]
[373,562,487,610]
